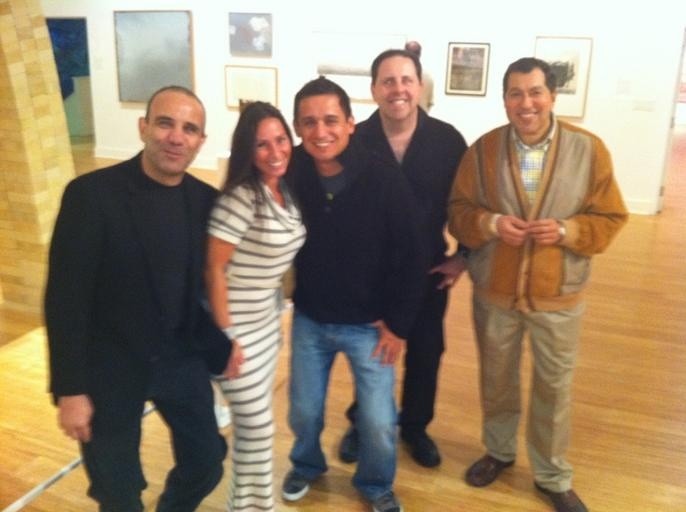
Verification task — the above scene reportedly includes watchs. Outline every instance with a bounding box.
[557,221,566,245]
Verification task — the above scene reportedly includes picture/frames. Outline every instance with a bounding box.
[224,65,278,112]
[534,36,593,122]
[114,10,194,105]
[445,42,490,96]
[229,12,272,59]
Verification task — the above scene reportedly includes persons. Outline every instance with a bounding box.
[448,58,629,512]
[205,102,307,512]
[340,51,471,467]
[405,42,434,115]
[43,86,231,512]
[282,76,426,512]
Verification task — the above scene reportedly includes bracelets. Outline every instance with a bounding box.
[220,326,237,339]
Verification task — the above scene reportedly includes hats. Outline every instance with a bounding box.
[534,482,587,512]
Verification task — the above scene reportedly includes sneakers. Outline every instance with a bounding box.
[282,472,309,501]
[371,493,403,512]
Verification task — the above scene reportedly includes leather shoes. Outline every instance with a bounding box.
[465,455,514,487]
[411,437,440,467]
[340,428,357,462]
